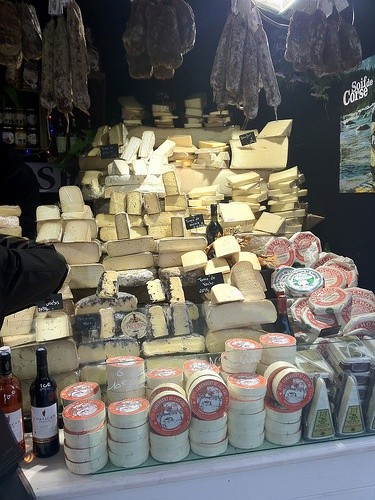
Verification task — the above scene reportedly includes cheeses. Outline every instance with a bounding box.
[0,93,326,413]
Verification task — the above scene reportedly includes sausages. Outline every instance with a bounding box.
[1,0,365,118]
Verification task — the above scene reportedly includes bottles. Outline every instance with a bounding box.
[55,112,79,154]
[0,346,25,454]
[29,348,59,458]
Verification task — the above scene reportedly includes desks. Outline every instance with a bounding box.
[15,425,375,499]
